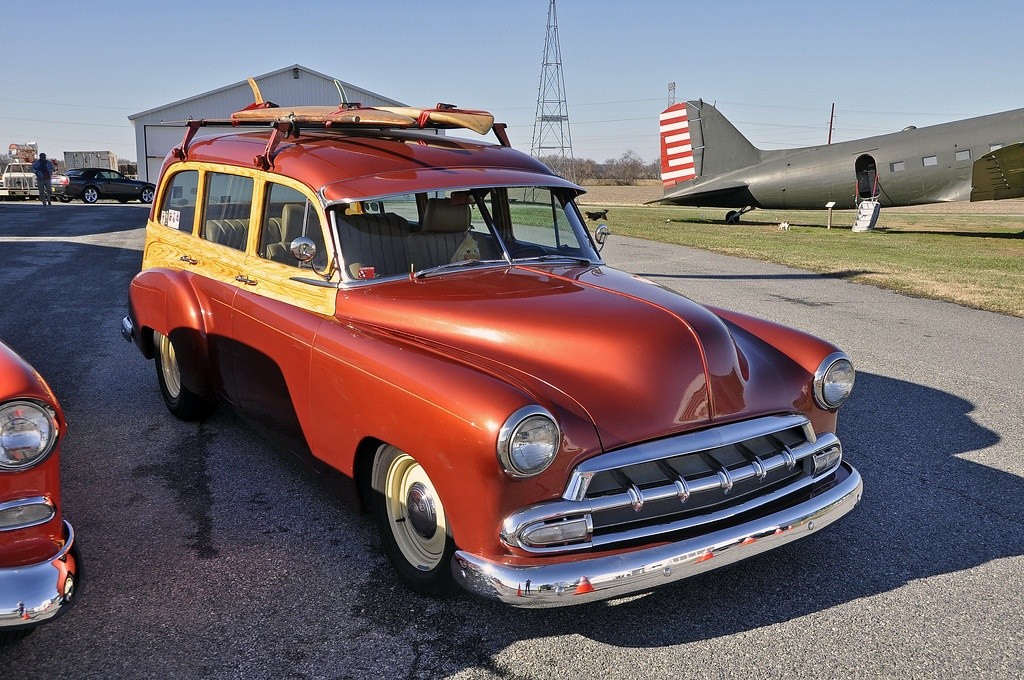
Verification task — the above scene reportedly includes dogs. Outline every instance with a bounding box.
[778,221,790,231]
[584,208,610,222]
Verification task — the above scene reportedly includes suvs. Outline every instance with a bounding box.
[120,74,864,610]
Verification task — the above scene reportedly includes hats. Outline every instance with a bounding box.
[39,153,47,156]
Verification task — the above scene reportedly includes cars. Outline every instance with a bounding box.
[0,337,84,636]
[51,167,157,205]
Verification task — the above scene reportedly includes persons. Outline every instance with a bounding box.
[32,153,53,206]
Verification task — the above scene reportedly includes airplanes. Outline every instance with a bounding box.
[641,97,1024,226]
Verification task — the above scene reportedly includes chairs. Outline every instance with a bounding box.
[97,173,105,178]
[205,198,496,270]
